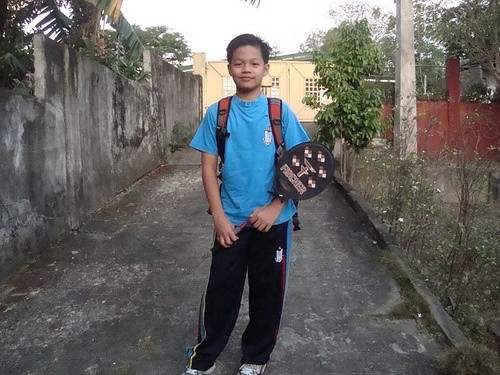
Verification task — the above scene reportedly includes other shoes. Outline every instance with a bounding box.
[182,363,215,375]
[238,363,266,375]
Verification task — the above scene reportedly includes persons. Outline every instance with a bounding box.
[182,34,310,375]
